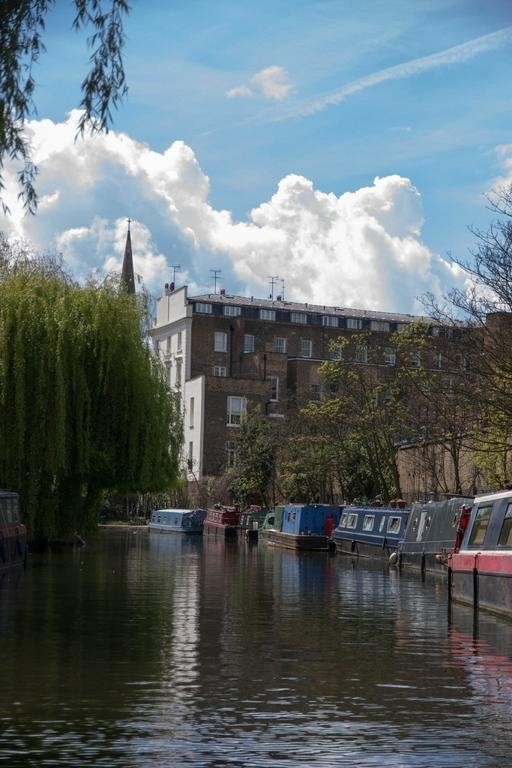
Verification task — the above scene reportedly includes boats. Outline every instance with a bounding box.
[0,491,85,583]
